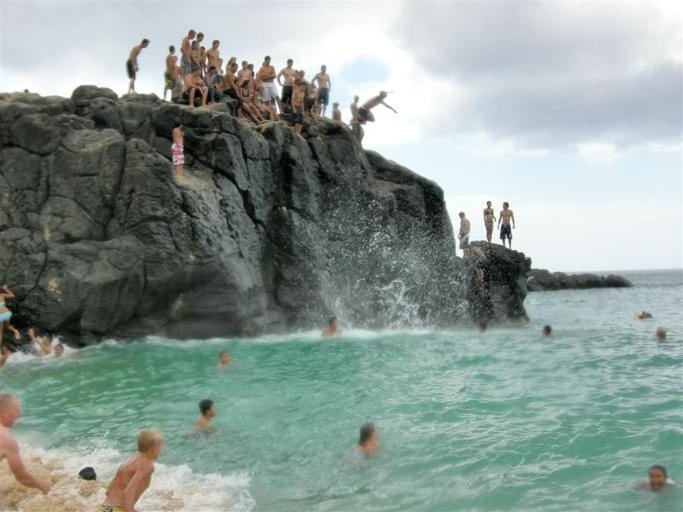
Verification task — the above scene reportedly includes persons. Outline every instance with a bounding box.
[464,245,488,283]
[540,323,554,336]
[124,37,148,90]
[653,327,665,341]
[215,350,231,367]
[631,461,680,495]
[458,211,472,249]
[347,421,379,466]
[1,390,52,496]
[478,321,486,330]
[168,119,187,178]
[483,200,498,241]
[77,466,99,479]
[166,29,396,147]
[102,428,165,511]
[632,309,654,321]
[498,202,516,247]
[0,284,64,367]
[194,399,219,435]
[320,315,345,339]
